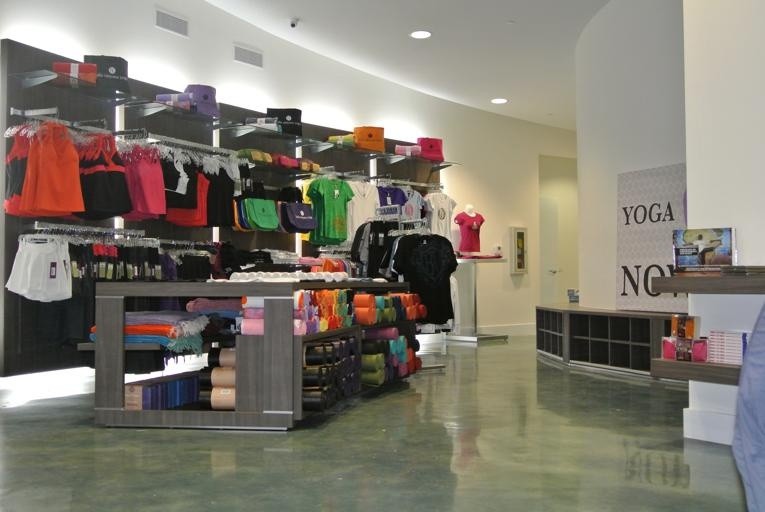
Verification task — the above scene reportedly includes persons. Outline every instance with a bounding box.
[454,204,485,256]
[673,234,679,270]
[683,234,722,266]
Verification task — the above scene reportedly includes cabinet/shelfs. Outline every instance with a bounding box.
[443,258,508,342]
[536,305,688,388]
[94,281,410,431]
[650,277,765,385]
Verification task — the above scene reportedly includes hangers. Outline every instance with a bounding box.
[311,166,445,199]
[4,109,241,170]
[365,204,431,238]
[18,222,214,251]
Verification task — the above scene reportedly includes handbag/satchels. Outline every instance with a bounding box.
[245,117,279,132]
[266,109,303,136]
[229,178,278,231]
[418,138,444,161]
[278,185,315,233]
[186,85,219,116]
[84,55,128,93]
[354,127,386,152]
[52,62,97,87]
[395,145,422,157]
[329,134,355,147]
[157,92,197,112]
